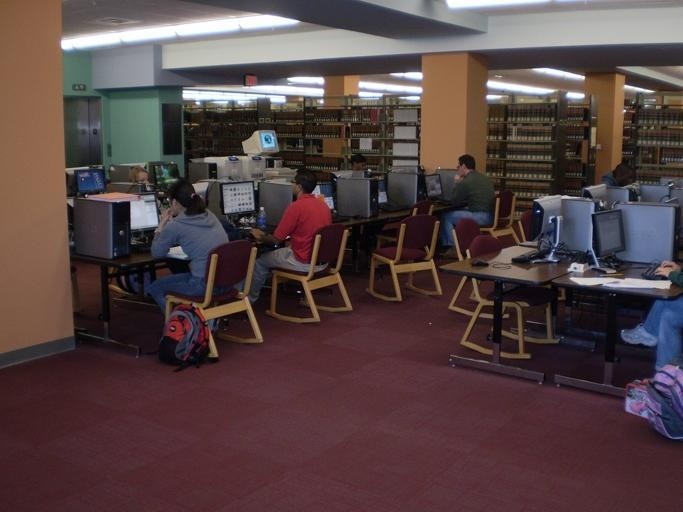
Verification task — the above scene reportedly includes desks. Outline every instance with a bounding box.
[335,199,466,273]
[438,245,570,385]
[70,248,167,357]
[550,261,682,398]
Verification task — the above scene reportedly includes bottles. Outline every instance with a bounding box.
[256,206,267,230]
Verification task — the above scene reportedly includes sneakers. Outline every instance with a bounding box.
[439,246,459,259]
[620,322,658,348]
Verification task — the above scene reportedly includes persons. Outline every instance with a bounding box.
[244,167,332,305]
[602,162,639,189]
[621,260,682,372]
[441,155,496,258]
[132,166,150,192]
[148,178,234,322]
[349,154,367,170]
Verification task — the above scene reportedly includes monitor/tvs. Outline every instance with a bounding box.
[582,184,607,202]
[220,181,257,215]
[241,129,279,157]
[65,159,187,196]
[592,209,626,272]
[313,182,336,212]
[529,194,562,264]
[126,192,160,239]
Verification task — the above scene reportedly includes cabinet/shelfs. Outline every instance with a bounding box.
[488,91,591,220]
[622,93,683,185]
[183,93,420,175]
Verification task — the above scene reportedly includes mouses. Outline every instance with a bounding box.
[472,259,489,266]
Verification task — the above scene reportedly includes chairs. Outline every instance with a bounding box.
[453,218,511,318]
[265,225,355,324]
[166,240,264,360]
[480,190,521,245]
[459,235,561,358]
[366,214,441,302]
[510,209,567,301]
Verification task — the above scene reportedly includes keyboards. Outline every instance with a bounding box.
[332,217,349,223]
[642,263,668,280]
[512,250,538,263]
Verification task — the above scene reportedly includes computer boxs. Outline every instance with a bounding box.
[258,181,297,225]
[540,195,603,257]
[73,198,130,261]
[608,176,682,263]
[335,166,461,219]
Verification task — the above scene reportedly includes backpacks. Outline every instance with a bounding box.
[646,364,682,440]
[155,304,210,368]
